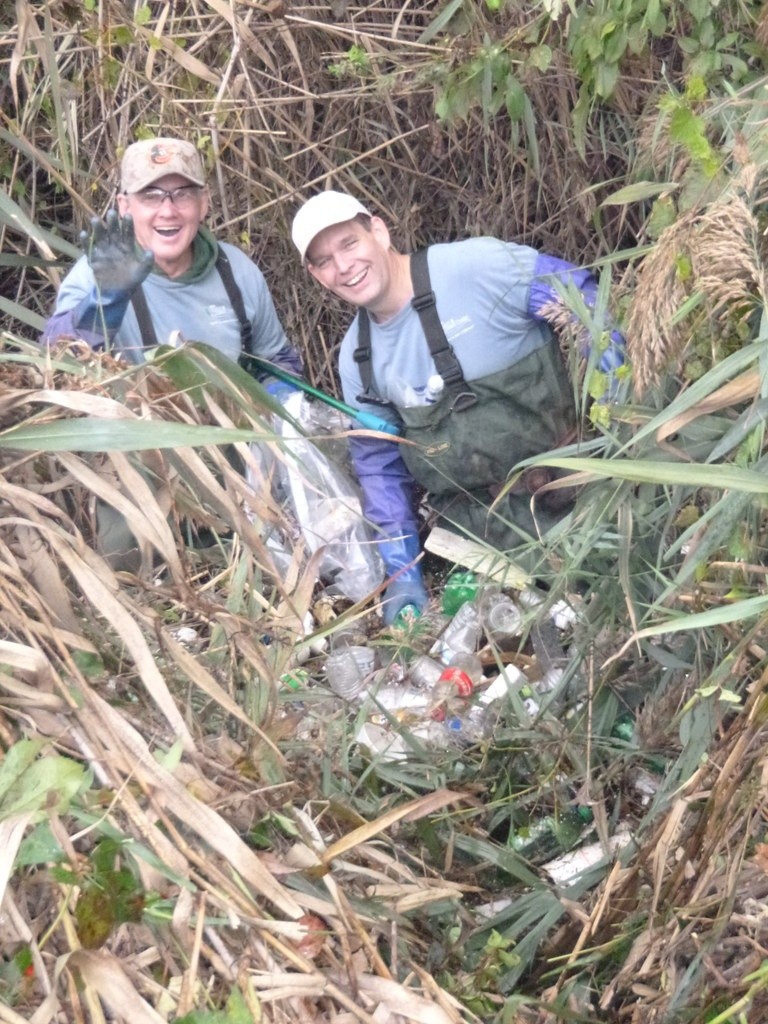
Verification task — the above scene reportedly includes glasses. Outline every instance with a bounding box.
[137,184,204,206]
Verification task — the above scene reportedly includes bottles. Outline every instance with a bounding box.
[422,373,444,407]
[264,569,667,884]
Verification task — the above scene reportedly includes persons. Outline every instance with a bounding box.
[41,138,310,582]
[290,191,626,625]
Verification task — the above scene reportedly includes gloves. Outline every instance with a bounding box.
[343,425,429,626]
[525,252,627,408]
[256,337,315,401]
[37,209,154,359]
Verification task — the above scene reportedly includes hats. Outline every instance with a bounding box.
[119,137,206,194]
[291,190,373,267]
